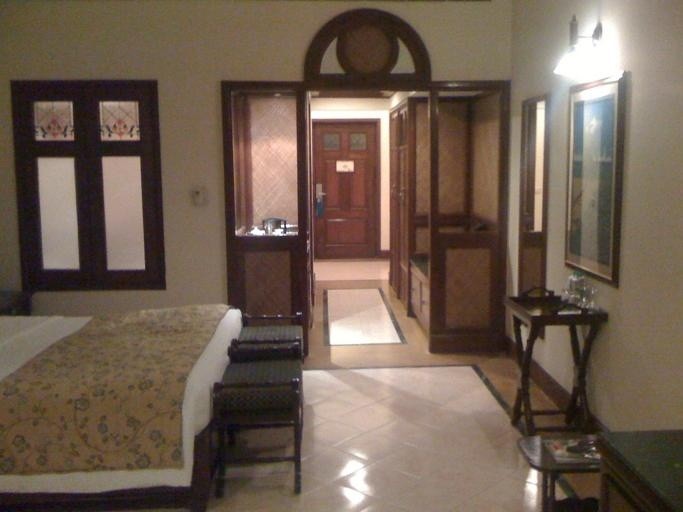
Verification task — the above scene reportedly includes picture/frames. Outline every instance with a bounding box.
[563,71,626,289]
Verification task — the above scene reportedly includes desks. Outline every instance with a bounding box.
[594,428,683,512]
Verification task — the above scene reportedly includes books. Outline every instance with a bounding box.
[544,434,601,466]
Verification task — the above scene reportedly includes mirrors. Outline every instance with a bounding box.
[519,92,549,340]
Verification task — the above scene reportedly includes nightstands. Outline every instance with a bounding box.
[0,289,35,315]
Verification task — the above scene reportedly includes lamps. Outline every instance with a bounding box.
[553,14,603,77]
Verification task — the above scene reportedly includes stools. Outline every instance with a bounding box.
[211,342,303,498]
[227,312,306,354]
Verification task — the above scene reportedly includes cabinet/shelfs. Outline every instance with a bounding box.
[389,105,408,308]
[409,260,429,332]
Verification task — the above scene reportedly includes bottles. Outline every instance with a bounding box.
[569,271,585,299]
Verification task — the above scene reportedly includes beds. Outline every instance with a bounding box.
[0,303,243,512]
